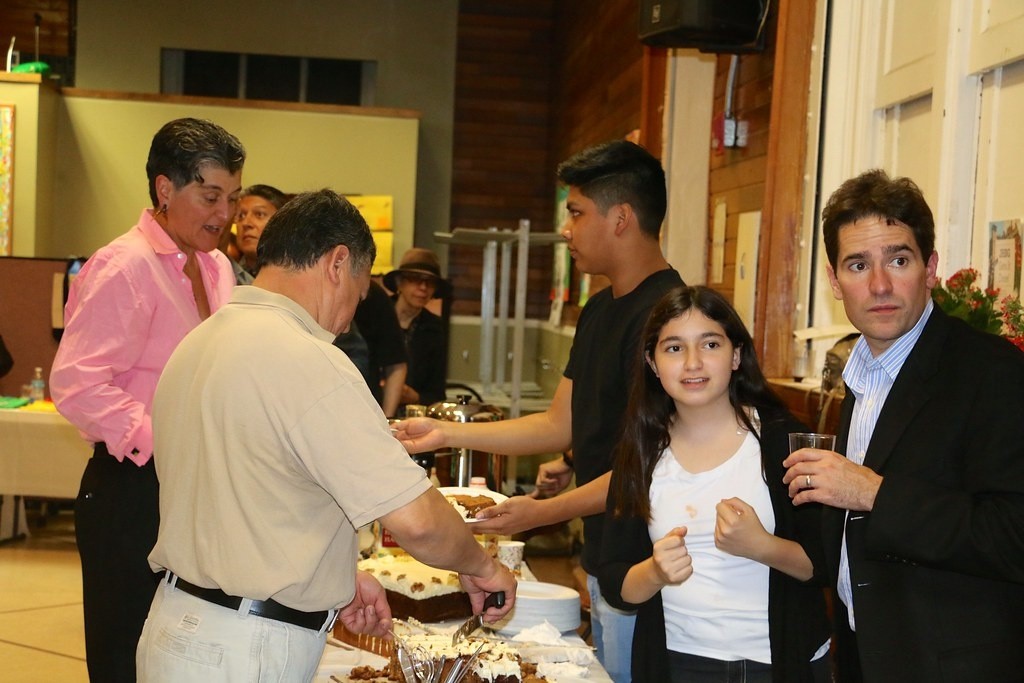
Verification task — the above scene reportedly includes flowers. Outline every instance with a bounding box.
[932,267,1024,352]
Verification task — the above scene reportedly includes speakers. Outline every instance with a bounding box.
[635,0,765,49]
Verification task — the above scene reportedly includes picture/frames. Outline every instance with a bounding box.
[987,218,1024,334]
[549,187,572,301]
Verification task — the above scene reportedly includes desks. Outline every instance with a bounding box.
[766,375,847,450]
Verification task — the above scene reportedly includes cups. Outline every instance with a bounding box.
[497,541,526,570]
[788,432,836,455]
[405,404,427,417]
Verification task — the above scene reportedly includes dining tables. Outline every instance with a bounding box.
[311,466,613,683]
[0,399,97,547]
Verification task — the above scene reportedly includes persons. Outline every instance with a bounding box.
[48,118,519,683]
[783,169,1024,683]
[387,139,687,683]
[597,284,831,683]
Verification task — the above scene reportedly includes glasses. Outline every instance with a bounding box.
[402,274,437,288]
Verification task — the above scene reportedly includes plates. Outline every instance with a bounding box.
[480,581,581,638]
[435,487,509,523]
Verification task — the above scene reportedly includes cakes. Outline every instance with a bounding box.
[355,552,472,624]
[443,494,498,520]
[350,633,551,683]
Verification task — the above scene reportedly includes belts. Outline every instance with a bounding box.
[165,570,337,633]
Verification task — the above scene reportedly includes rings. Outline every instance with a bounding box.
[806,474,811,489]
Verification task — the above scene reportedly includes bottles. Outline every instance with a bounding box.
[468,477,490,490]
[429,466,441,488]
[30,366,45,401]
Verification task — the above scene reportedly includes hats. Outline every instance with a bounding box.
[383,248,453,299]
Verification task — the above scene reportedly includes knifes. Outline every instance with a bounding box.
[397,648,417,683]
[452,591,506,648]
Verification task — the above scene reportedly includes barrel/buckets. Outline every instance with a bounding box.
[426,394,505,493]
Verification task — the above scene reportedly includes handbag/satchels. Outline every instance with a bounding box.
[822,331,862,397]
